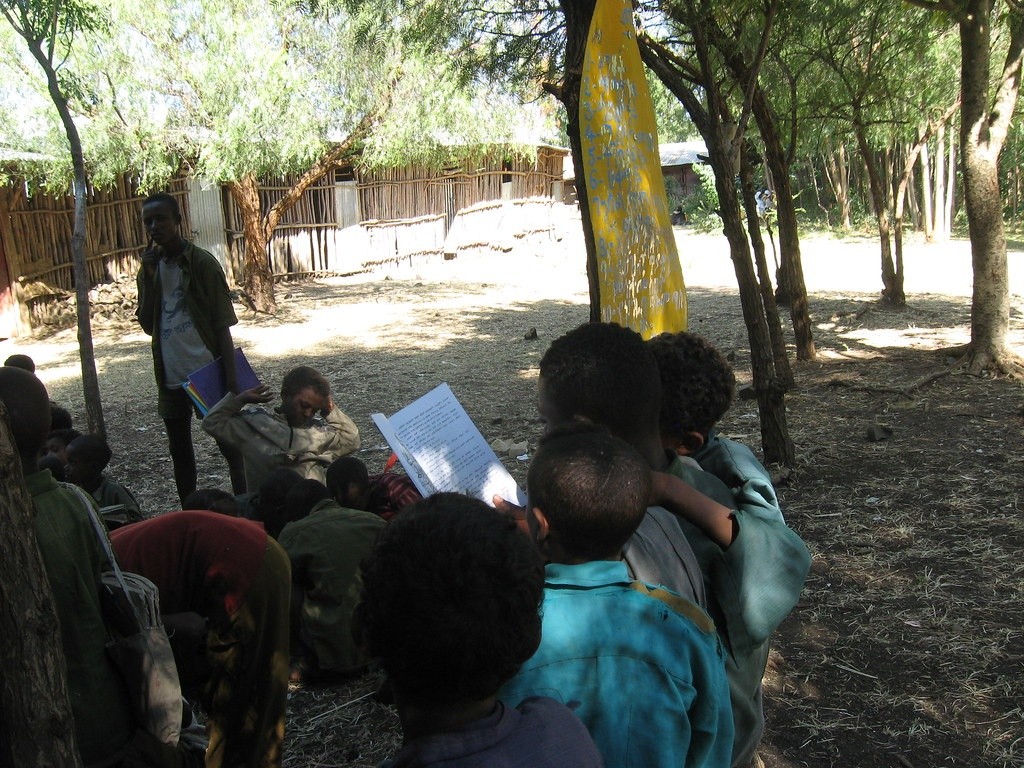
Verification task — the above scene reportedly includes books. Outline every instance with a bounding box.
[372,382,528,511]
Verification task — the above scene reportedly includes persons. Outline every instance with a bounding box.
[135,193,246,512]
[0,323,771,768]
[646,331,809,766]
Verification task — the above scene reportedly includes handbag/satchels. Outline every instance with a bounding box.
[61,482,182,746]
